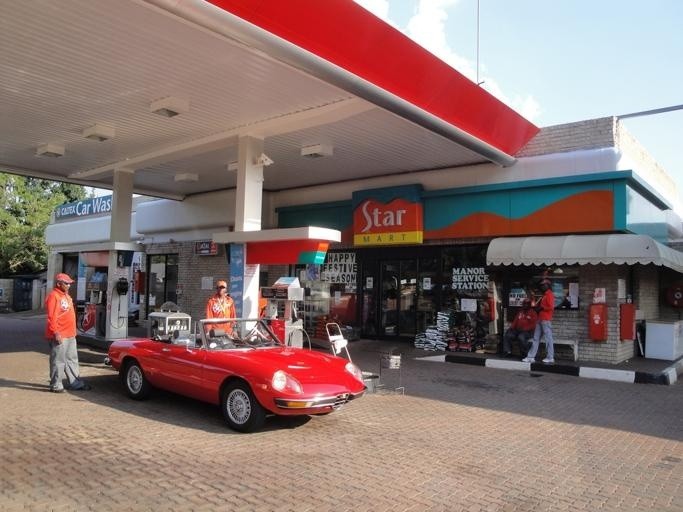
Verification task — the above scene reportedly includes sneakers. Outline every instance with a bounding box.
[50,384,91,393]
[502,353,555,363]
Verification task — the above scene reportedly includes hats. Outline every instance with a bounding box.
[55,273,75,284]
[523,298,530,303]
[540,279,551,284]
[217,280,227,288]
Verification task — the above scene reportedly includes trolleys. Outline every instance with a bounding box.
[325,322,380,394]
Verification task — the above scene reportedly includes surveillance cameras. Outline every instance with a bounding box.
[261,152,274,167]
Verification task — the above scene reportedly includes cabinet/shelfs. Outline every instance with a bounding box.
[644,317,683,361]
[148,312,191,338]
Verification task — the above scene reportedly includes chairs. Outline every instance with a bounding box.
[168,330,200,347]
[206,328,235,348]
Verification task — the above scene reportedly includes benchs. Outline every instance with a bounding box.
[524,338,578,363]
[152,333,207,345]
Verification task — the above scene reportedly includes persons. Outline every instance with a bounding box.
[521,280,556,363]
[44,273,93,393]
[203,280,239,339]
[498,297,537,359]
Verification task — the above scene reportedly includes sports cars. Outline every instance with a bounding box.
[105,318,368,433]
